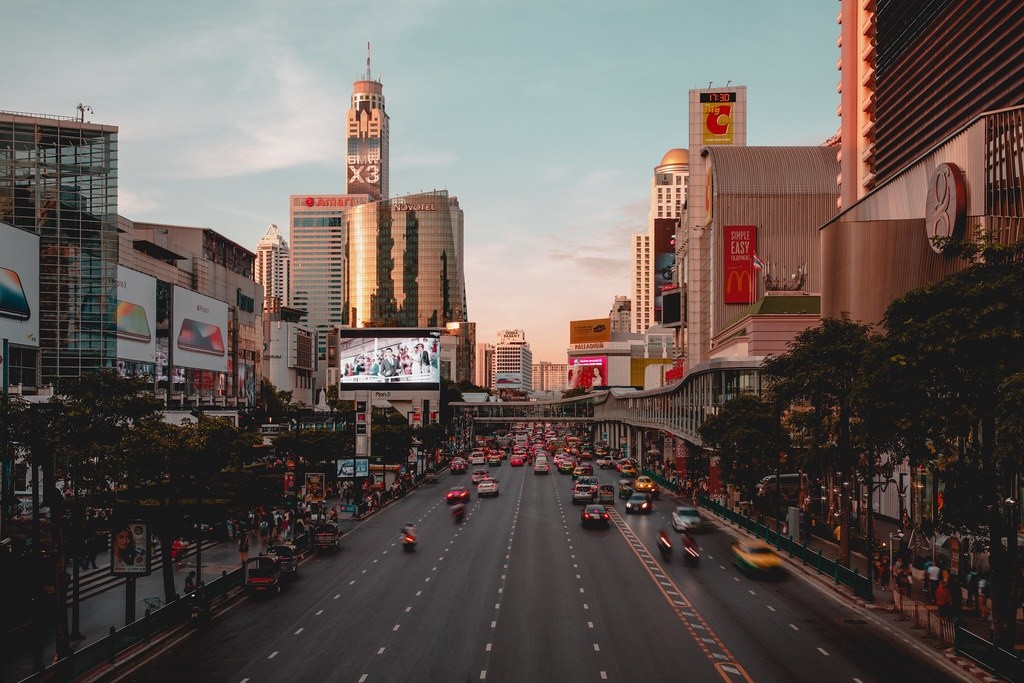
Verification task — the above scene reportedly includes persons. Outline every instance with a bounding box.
[343,343,430,382]
[834,524,841,544]
[114,524,137,566]
[151,533,159,558]
[227,480,386,561]
[171,538,182,566]
[902,508,909,532]
[399,522,416,540]
[568,369,574,388]
[409,448,416,461]
[872,551,994,630]
[85,535,99,569]
[592,367,602,387]
[184,571,195,595]
[390,470,415,498]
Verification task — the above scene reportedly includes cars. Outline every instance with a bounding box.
[445,422,659,505]
[671,506,704,534]
[625,492,653,515]
[579,504,610,530]
[728,538,784,580]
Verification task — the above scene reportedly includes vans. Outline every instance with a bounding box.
[243,555,282,593]
[754,472,810,506]
[314,522,340,551]
[267,544,299,577]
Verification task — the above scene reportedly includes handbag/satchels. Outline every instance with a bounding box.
[278,515,282,524]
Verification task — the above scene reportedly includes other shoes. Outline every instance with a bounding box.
[875,580,879,585]
[881,586,885,590]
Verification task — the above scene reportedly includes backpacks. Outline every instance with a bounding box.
[261,521,268,537]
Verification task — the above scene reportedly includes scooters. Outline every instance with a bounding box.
[655,531,672,553]
[450,499,467,523]
[189,580,212,627]
[681,537,703,561]
[399,528,417,552]
